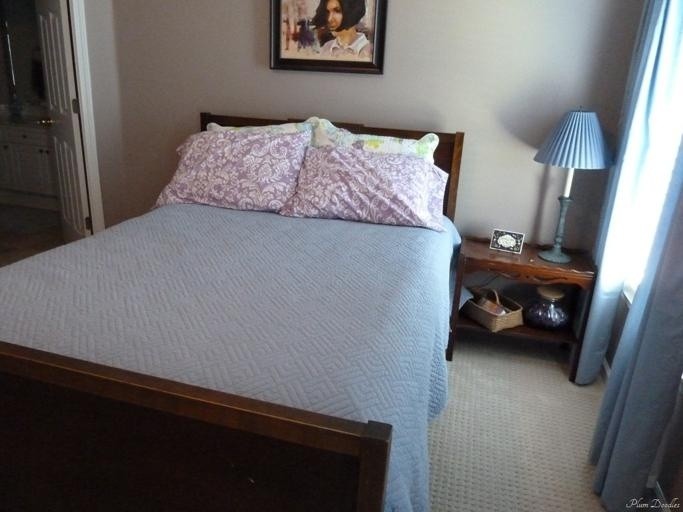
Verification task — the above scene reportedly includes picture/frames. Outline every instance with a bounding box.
[269,0,388,73]
[489,229,525,255]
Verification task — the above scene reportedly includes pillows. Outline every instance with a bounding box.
[158,117,448,232]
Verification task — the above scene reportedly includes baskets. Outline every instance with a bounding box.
[461,287,525,333]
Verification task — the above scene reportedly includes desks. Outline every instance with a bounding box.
[445,236,597,383]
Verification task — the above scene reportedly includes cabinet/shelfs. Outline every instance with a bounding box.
[0,122,61,211]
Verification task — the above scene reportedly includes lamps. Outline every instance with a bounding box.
[535,106,615,263]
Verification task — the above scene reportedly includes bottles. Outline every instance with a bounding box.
[528,287,566,328]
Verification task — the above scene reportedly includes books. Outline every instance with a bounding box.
[474,296,506,314]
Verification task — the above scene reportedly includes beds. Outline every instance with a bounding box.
[0,113,464,511]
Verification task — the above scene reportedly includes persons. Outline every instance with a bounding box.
[311,1,372,59]
